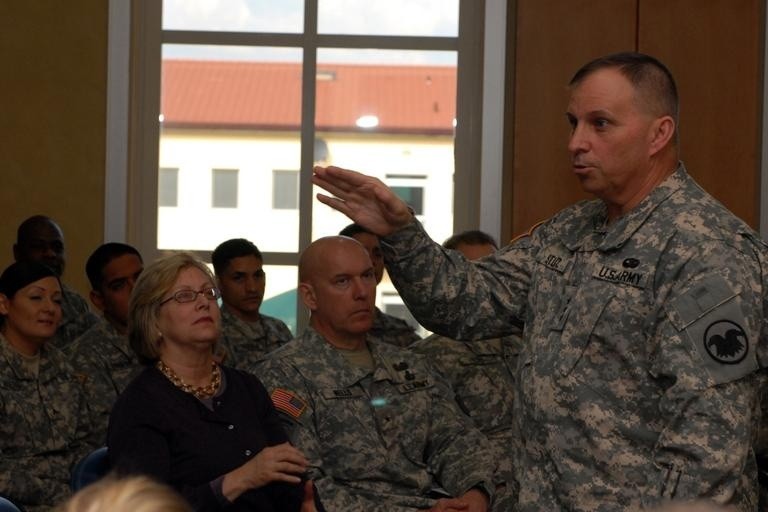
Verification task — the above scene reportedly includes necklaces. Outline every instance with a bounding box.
[157,356,225,400]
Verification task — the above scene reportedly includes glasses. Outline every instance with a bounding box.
[161,286,221,303]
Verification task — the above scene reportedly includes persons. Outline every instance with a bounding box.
[59,244,146,403]
[233,234,504,509]
[0,258,123,512]
[104,249,311,512]
[12,214,92,355]
[204,237,293,378]
[307,50,766,511]
[337,222,386,287]
[441,229,501,263]
